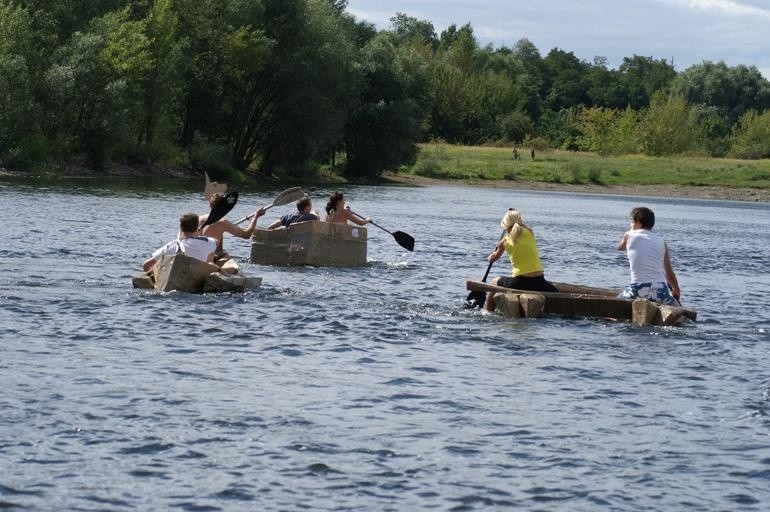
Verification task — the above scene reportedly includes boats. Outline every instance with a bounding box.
[492,272,697,330]
[250,223,368,268]
[132,253,264,293]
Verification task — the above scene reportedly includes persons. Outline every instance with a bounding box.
[267,196,321,229]
[616,206,683,308]
[530,143,536,162]
[486,207,560,293]
[141,212,222,273]
[197,191,268,275]
[324,190,374,228]
[512,147,517,160]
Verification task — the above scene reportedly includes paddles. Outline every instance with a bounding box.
[196,191,239,232]
[464,207,515,308]
[233,187,303,226]
[346,206,414,252]
